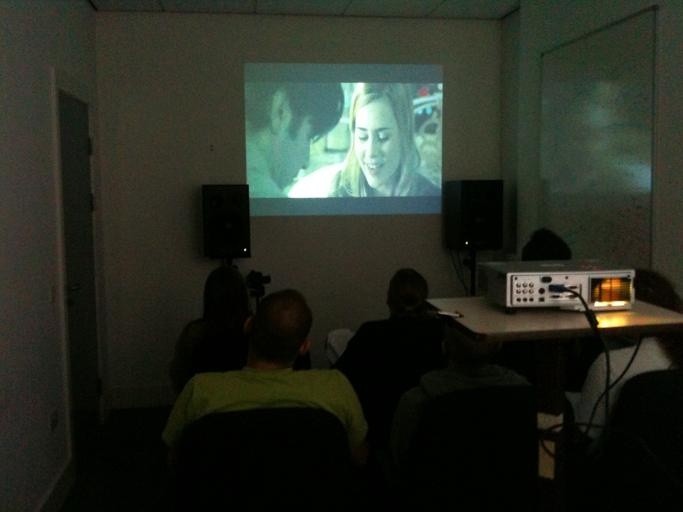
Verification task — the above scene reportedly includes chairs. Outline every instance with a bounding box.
[407,383,573,512]
[173,407,354,511]
[567,367,683,511]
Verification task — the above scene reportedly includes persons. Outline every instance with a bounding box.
[384,320,534,488]
[243,80,345,200]
[325,267,495,364]
[162,287,367,464]
[329,81,441,199]
[518,228,570,261]
[170,265,251,393]
[575,267,683,439]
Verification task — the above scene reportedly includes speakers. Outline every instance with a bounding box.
[442,178,504,255]
[201,183,250,259]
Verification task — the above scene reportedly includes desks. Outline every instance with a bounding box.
[427,295,683,366]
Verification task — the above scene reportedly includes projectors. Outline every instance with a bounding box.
[479,259,637,314]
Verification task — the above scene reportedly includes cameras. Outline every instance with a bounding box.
[246,269,270,297]
